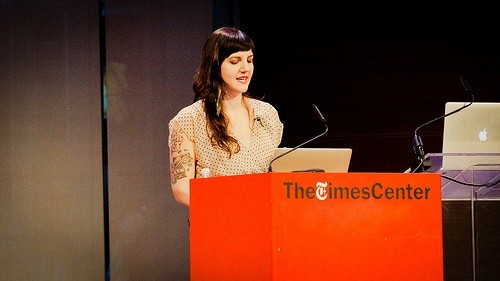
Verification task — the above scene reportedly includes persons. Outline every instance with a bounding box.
[167,26,283,207]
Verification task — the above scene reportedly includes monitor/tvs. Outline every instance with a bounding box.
[271,148,352,173]
[441,102,500,170]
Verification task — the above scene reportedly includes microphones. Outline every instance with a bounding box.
[410,76,475,173]
[267,103,328,172]
